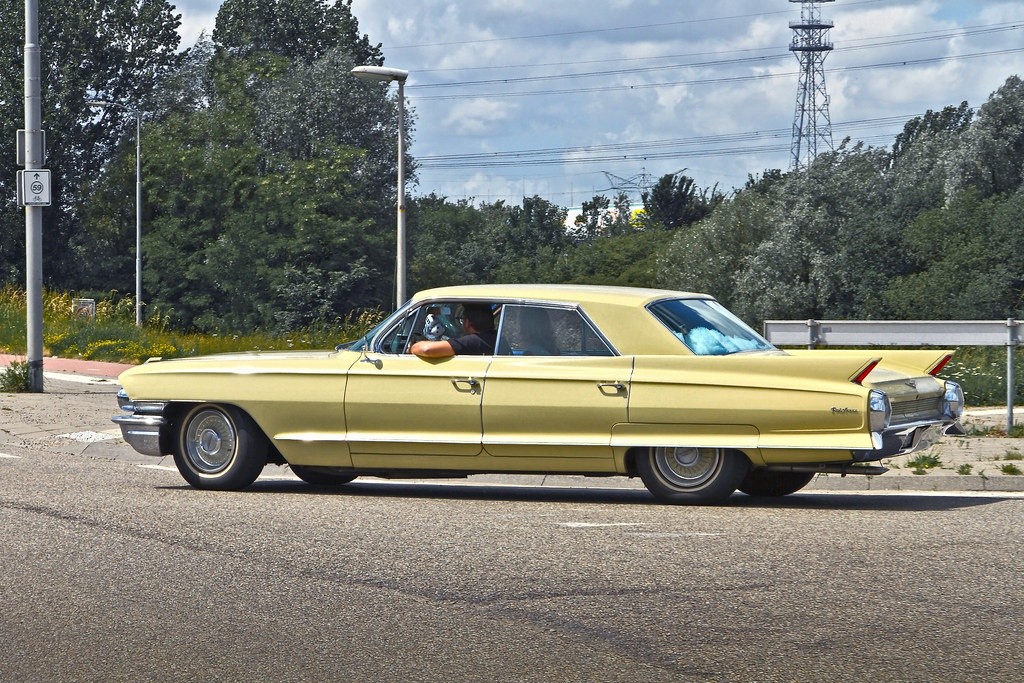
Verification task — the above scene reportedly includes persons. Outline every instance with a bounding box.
[517,307,561,356]
[411,302,514,357]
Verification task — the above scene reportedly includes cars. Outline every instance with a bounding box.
[112,285,965,506]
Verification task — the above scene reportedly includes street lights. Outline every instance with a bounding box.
[86,101,148,329]
[350,66,410,335]
